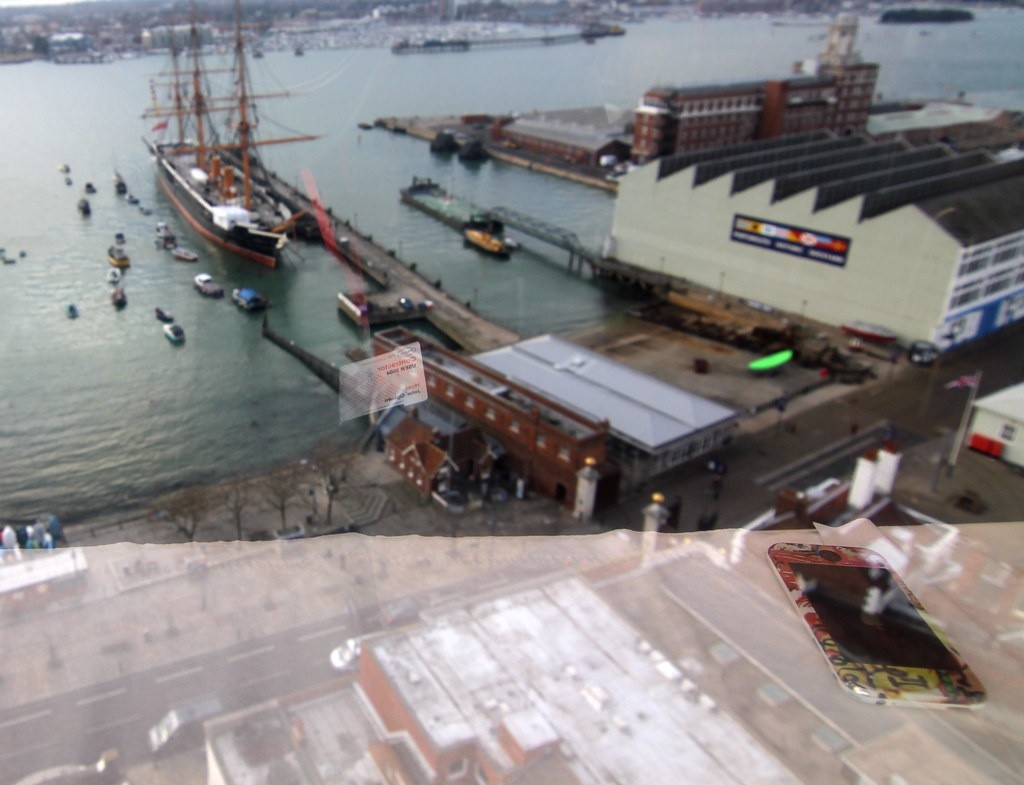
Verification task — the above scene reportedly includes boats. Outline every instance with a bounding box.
[194,274,223,296]
[232,288,265,310]
[0,160,200,322]
[465,229,508,259]
[162,324,185,344]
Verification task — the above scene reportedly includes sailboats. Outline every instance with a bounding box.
[141,1,321,270]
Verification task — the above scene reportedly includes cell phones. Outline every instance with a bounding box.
[767,540,988,705]
